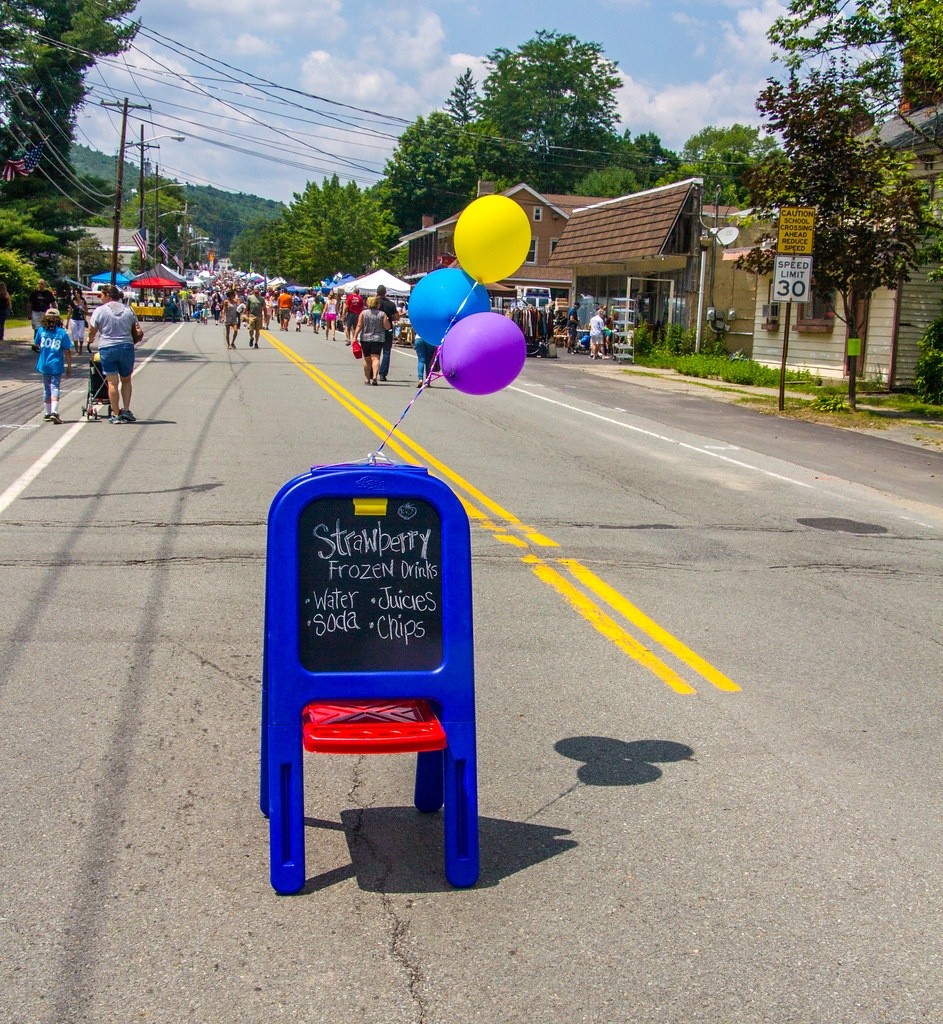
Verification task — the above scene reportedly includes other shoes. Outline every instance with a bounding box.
[249,338,254,346]
[380,376,386,381]
[417,381,423,388]
[231,344,236,348]
[255,344,258,348]
[372,379,377,385]
[365,381,369,384]
[228,346,231,349]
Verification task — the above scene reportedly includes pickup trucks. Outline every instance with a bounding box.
[70,282,128,307]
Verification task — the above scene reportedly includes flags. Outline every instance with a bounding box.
[187,259,207,271]
[2,140,45,182]
[172,248,184,270]
[131,227,146,260]
[158,237,168,265]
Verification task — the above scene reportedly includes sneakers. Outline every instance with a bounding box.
[44,414,52,421]
[50,413,62,424]
[109,415,121,423]
[122,411,136,421]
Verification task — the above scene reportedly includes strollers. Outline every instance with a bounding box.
[80,341,136,420]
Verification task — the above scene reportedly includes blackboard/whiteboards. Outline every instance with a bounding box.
[264,466,476,704]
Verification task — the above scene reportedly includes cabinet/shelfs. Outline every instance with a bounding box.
[613,298,635,364]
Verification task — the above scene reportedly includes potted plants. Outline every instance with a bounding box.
[763,304,778,320]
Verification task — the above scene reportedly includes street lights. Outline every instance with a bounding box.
[110,133,185,286]
[694,230,711,356]
[139,183,214,306]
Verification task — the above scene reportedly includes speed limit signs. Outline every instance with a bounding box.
[772,255,816,303]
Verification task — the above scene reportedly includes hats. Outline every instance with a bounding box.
[45,308,60,316]
[377,285,386,294]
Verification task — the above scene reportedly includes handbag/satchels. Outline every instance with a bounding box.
[353,340,362,359]
[129,306,143,344]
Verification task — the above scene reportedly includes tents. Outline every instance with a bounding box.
[232,268,411,309]
[91,262,218,303]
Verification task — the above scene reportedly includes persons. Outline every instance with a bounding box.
[28,278,59,351]
[87,285,144,424]
[588,305,619,360]
[376,285,399,381]
[66,288,88,355]
[567,303,581,355]
[354,296,391,386]
[31,308,74,424]
[0,281,12,341]
[553,311,567,328]
[161,270,371,349]
[414,333,435,388]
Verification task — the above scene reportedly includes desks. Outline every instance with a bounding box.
[395,324,413,348]
[130,306,164,321]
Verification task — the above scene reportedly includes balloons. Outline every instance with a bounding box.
[408,268,490,348]
[439,312,526,395]
[454,194,531,285]
